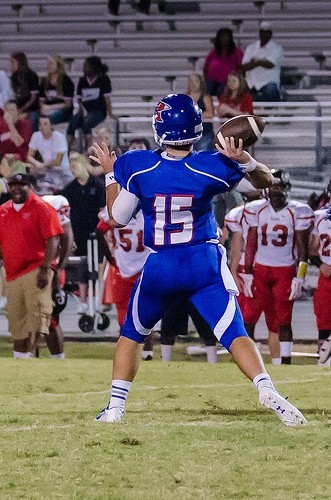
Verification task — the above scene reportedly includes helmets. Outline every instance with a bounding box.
[153,93,203,146]
[269,168,291,191]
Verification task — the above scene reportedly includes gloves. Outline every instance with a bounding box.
[239,273,254,298]
[289,276,305,300]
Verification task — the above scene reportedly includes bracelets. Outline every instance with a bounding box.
[39,265,47,272]
[238,159,256,173]
[105,172,117,187]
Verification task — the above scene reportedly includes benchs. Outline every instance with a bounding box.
[0,0,331,164]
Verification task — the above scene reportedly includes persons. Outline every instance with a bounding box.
[225,170,316,364]
[27,174,74,359]
[160,301,217,364]
[95,183,153,362]
[0,173,64,358]
[186,22,283,150]
[0,49,152,315]
[89,94,306,428]
[308,180,331,363]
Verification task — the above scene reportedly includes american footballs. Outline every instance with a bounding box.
[213,114,265,150]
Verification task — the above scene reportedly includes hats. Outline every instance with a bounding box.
[7,173,30,185]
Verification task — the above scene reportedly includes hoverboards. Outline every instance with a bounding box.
[78,232,110,333]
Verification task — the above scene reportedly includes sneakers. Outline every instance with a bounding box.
[258,385,307,427]
[93,403,122,423]
